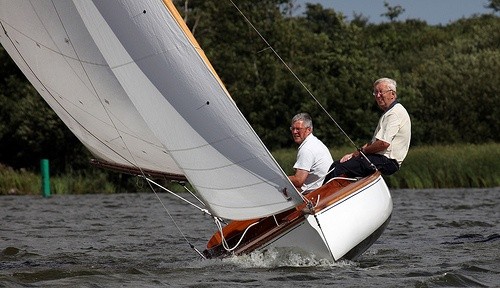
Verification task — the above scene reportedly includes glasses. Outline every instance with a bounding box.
[373,89,393,95]
[290,126,309,130]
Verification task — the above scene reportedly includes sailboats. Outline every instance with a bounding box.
[0,0,394,268]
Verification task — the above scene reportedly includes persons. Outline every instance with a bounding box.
[288,114,333,196]
[323,78,412,186]
